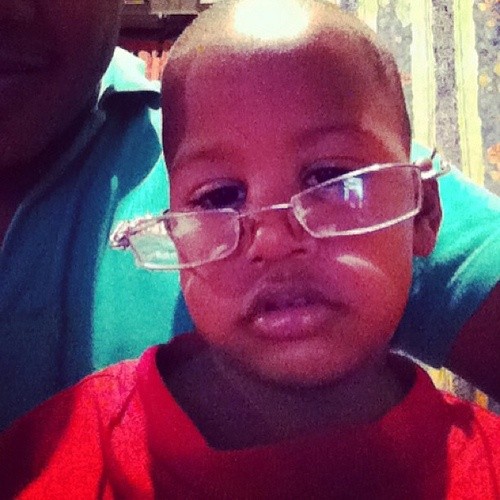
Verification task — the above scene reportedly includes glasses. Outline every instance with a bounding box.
[113,153,456,270]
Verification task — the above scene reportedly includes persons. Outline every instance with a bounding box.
[0,1,500,500]
